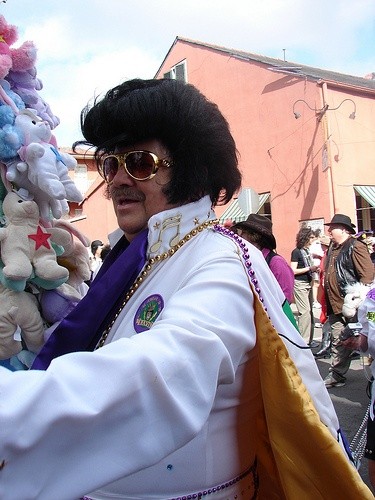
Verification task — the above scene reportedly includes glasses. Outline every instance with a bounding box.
[101,149,180,187]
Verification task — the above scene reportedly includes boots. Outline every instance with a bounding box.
[313,331,332,361]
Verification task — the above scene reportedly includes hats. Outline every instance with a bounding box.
[323,213,356,235]
[236,213,277,250]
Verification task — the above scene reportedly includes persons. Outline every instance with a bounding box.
[85,238,112,281]
[356,285,375,490]
[230,213,375,390]
[0,78,375,500]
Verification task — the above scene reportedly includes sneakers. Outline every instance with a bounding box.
[322,370,346,389]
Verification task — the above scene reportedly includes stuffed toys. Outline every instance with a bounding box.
[0,12,92,361]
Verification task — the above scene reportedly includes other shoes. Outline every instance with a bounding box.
[307,340,320,348]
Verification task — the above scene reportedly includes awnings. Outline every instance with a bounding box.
[354,186,375,209]
[218,193,271,227]
